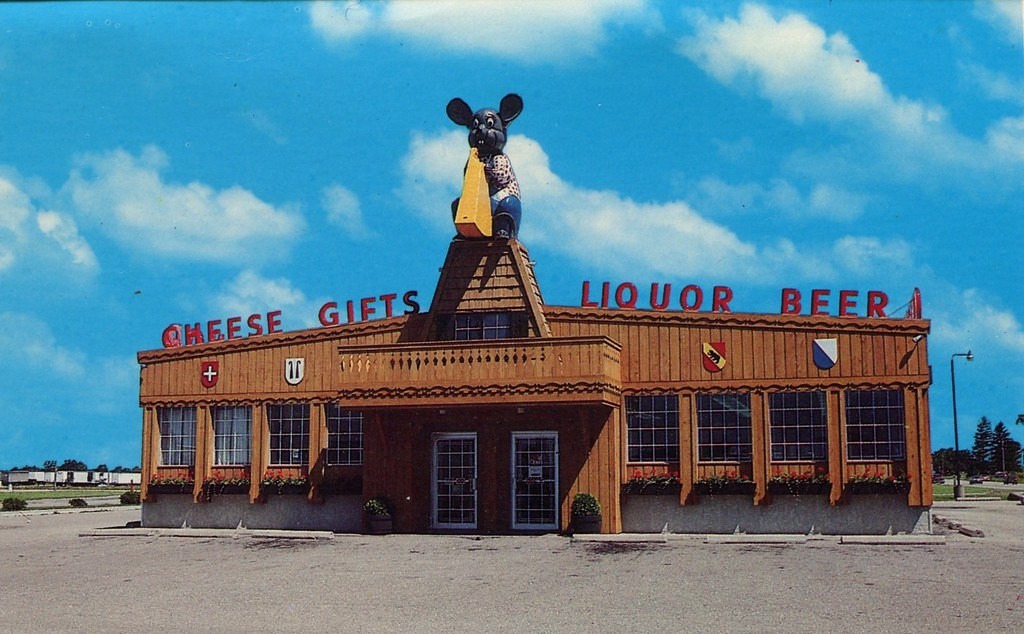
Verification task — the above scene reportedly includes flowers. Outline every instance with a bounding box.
[203,467,250,486]
[149,471,194,484]
[627,468,682,491]
[850,466,912,485]
[694,469,753,488]
[261,469,308,485]
[770,466,831,485]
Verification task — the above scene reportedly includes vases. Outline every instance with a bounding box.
[260,485,309,495]
[694,483,757,494]
[769,482,833,495]
[628,482,683,495]
[845,482,912,495]
[203,483,251,496]
[147,483,194,495]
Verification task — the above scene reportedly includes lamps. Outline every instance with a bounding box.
[912,335,923,344]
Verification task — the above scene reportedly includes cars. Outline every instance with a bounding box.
[932,473,946,484]
[982,476,990,480]
[968,476,983,485]
[1003,474,1018,486]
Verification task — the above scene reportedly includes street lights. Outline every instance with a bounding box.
[951,349,975,498]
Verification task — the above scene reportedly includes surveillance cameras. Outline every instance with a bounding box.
[967,354,973,360]
[912,335,922,342]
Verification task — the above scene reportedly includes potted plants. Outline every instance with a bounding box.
[571,493,603,534]
[363,496,395,534]
